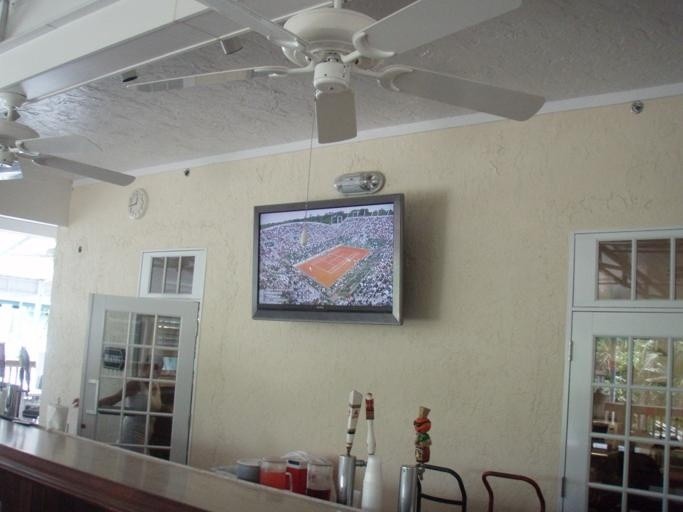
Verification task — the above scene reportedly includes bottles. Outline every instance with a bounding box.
[237,456,418,512]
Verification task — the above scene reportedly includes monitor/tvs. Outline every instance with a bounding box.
[251,193,404,326]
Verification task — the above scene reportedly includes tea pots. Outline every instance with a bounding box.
[47,403,68,432]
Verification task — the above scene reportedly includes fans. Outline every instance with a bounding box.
[0,84,133,186]
[121,0,549,145]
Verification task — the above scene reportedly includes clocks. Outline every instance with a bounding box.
[125,187,148,220]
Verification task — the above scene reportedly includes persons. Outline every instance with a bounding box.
[71,354,173,456]
[18,346,31,392]
[594,386,609,420]
